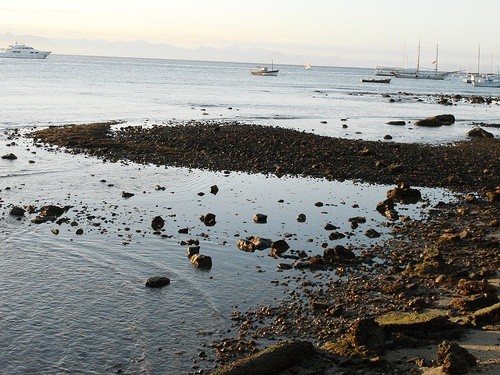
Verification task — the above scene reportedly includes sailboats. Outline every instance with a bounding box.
[462,43,500,87]
[390,42,450,80]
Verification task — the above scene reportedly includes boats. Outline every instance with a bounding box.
[374,66,418,75]
[362,78,392,84]
[0,41,52,59]
[251,59,279,76]
